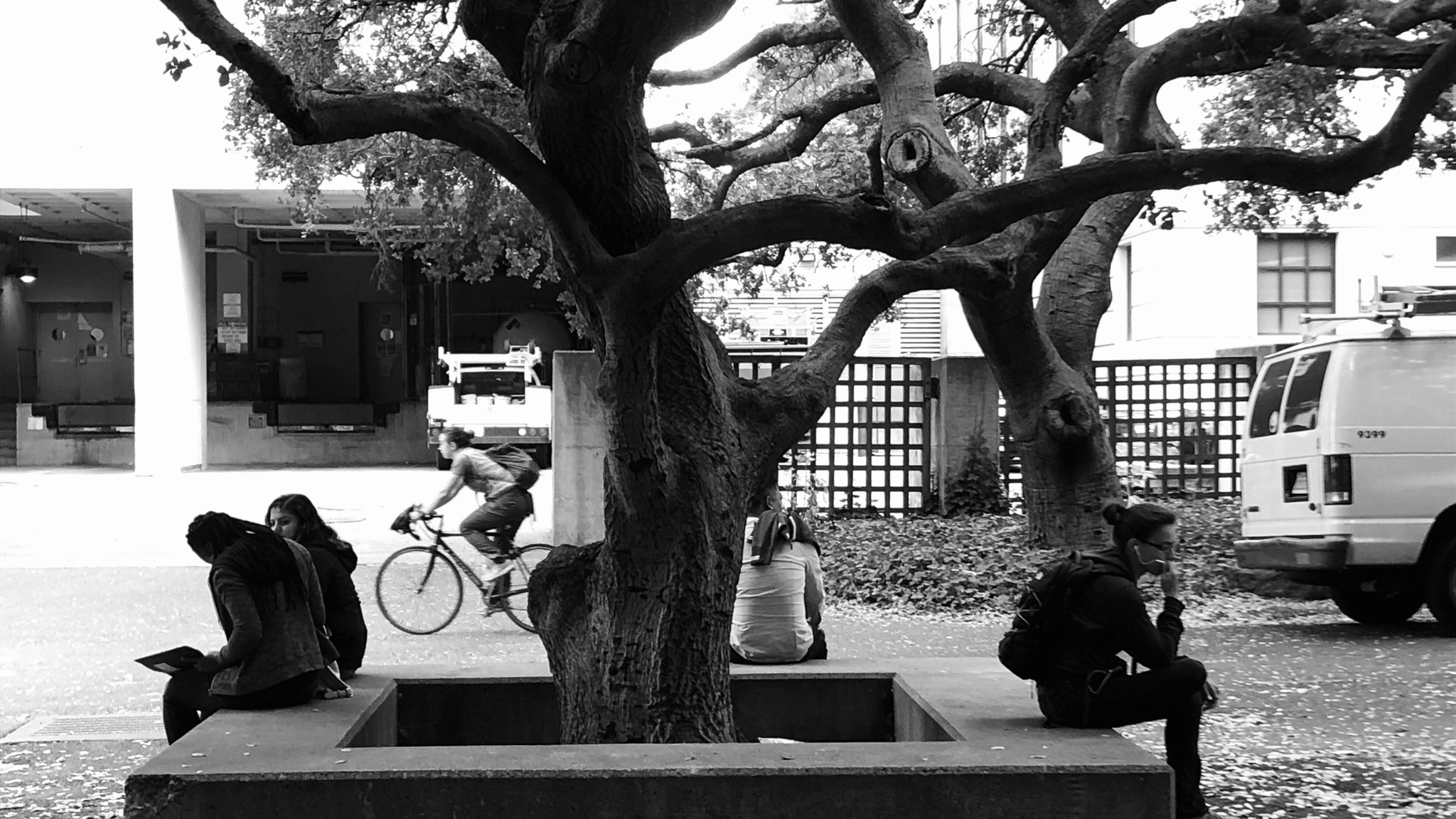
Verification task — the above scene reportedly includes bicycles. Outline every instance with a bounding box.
[376,508,554,635]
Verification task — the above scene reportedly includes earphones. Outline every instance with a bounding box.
[1133,545,1139,554]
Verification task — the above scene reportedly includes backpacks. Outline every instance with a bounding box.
[482,441,540,490]
[998,553,1137,684]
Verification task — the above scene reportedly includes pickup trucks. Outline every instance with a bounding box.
[424,346,554,472]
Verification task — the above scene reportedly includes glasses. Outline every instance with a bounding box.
[1145,541,1174,553]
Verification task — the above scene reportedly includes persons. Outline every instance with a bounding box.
[729,474,828,666]
[1036,503,1247,819]
[410,427,534,615]
[163,494,367,746]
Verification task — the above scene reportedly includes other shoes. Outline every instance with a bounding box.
[477,602,506,615]
[480,559,515,582]
[1194,810,1241,819]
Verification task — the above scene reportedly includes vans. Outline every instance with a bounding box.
[1230,313,1456,633]
